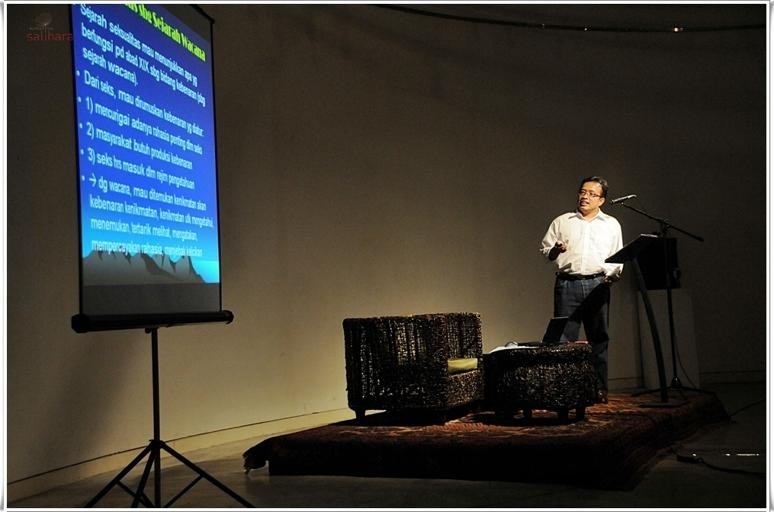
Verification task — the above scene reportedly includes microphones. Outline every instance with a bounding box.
[611,194,637,204]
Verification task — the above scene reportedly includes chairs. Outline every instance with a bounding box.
[342,308,482,422]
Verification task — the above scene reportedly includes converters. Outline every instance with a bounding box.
[677,452,702,464]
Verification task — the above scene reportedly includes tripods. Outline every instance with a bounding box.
[620,201,716,400]
[84,326,255,508]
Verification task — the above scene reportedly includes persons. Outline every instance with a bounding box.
[540,176,624,403]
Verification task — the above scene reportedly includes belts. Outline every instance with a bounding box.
[556,273,605,281]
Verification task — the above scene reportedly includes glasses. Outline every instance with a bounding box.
[577,190,602,198]
